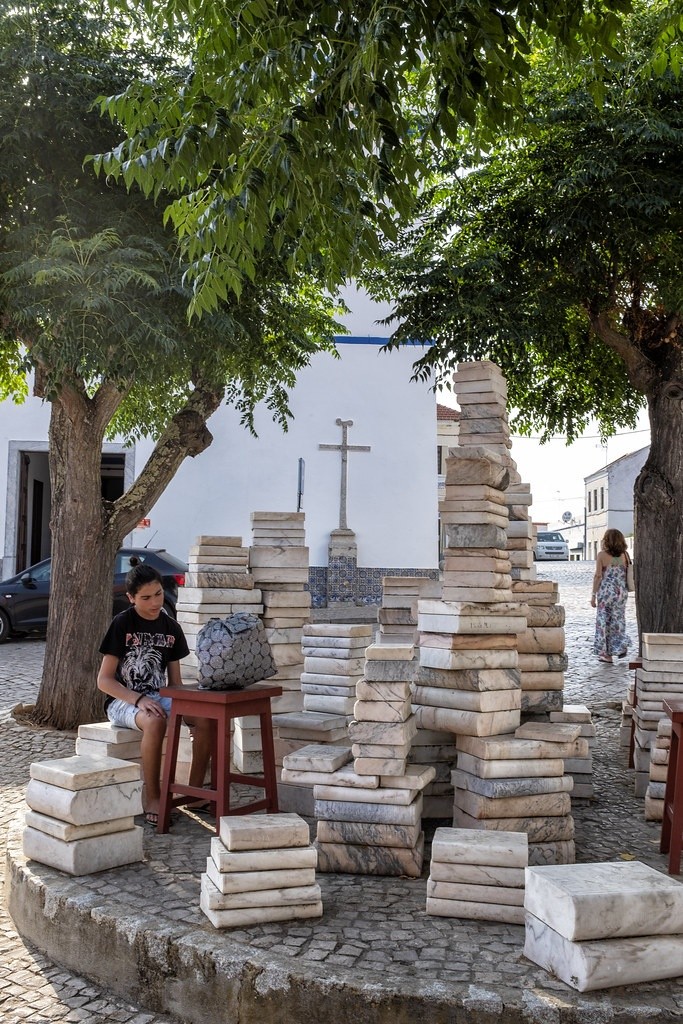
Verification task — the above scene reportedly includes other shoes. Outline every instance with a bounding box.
[618,648,627,659]
[601,656,612,662]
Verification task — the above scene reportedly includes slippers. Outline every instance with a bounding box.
[144,811,159,825]
[184,802,211,814]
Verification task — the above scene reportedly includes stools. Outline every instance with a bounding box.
[658,699,683,875]
[156,682,283,837]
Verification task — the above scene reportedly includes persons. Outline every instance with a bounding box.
[97,554,213,826]
[591,528,632,663]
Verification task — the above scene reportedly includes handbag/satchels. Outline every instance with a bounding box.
[625,551,635,592]
[194,612,279,690]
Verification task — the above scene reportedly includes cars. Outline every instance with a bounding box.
[533,530,569,561]
[1,546,188,645]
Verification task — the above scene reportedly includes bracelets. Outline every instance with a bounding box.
[135,693,147,707]
[592,594,596,597]
